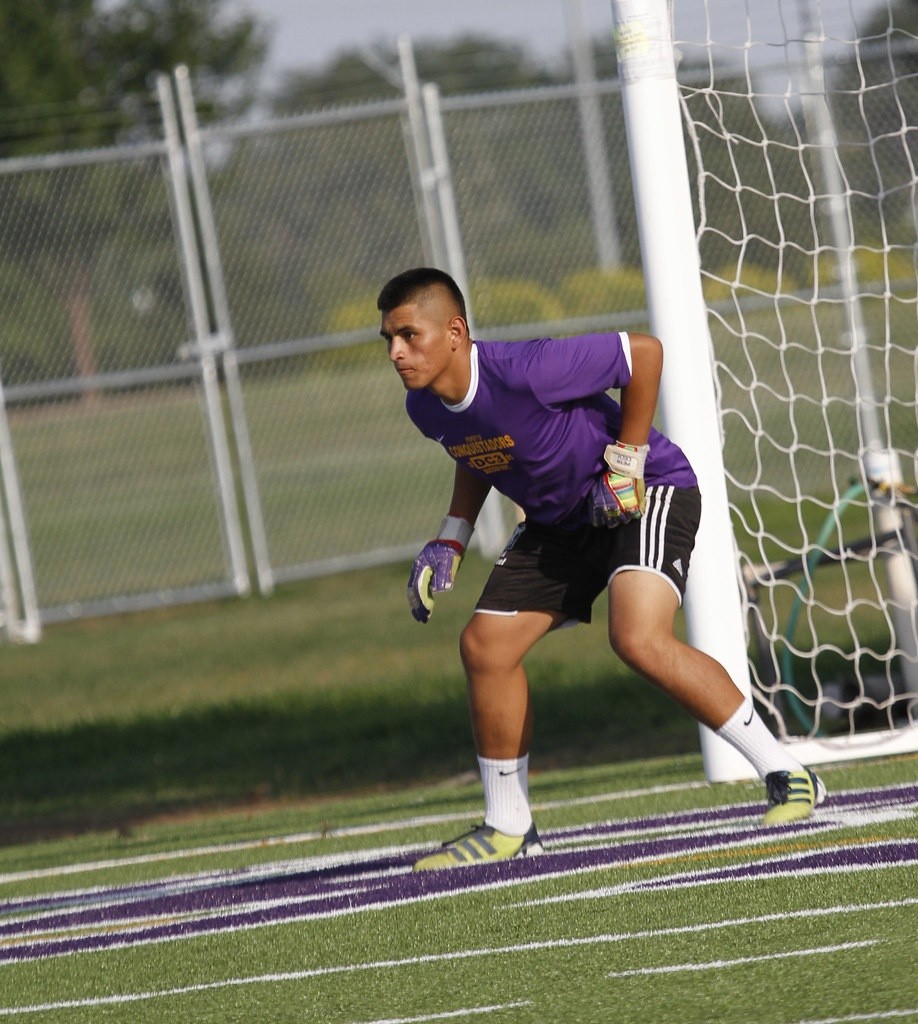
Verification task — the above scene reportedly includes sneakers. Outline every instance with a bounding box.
[763,762,827,824]
[412,820,544,872]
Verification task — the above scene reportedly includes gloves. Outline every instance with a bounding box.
[406,512,474,624]
[588,440,651,528]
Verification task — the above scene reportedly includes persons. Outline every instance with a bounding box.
[376,268,827,868]
